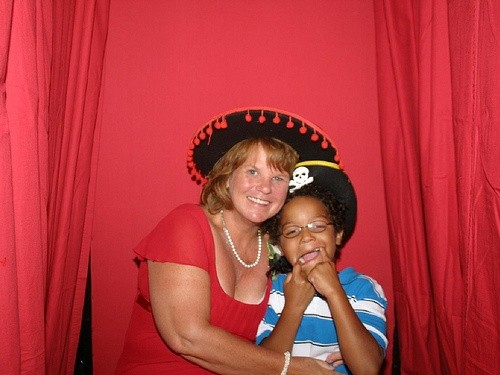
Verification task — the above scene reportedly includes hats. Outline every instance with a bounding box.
[285,156,359,246]
[185,104,338,185]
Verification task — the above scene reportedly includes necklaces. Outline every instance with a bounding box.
[221,210,261,268]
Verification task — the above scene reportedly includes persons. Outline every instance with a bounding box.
[114,108,339,375]
[256,161,388,375]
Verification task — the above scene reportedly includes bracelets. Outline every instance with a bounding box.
[281,351,290,375]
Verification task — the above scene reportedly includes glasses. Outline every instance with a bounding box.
[276,220,336,240]
